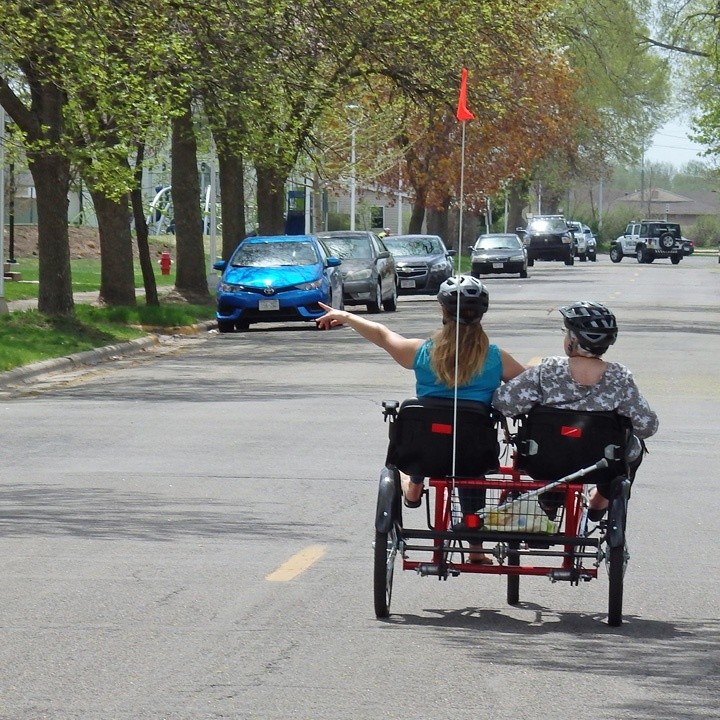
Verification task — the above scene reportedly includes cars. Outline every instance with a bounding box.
[382,235,457,295]
[467,234,528,279]
[681,237,694,256]
[316,231,399,313]
[212,236,345,332]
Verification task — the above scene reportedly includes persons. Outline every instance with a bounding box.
[378,228,390,239]
[315,275,525,564]
[490,301,659,522]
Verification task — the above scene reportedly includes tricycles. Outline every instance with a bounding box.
[374,397,649,627]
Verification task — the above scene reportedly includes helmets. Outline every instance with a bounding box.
[437,275,489,325]
[559,300,618,355]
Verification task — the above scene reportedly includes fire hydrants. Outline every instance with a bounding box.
[158,251,174,275]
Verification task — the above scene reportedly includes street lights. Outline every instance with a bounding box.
[345,105,361,231]
[3,112,20,264]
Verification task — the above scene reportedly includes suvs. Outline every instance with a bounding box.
[516,216,597,267]
[610,221,683,264]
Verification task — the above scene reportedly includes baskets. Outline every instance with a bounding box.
[445,475,569,536]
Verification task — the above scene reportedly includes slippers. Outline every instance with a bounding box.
[466,555,493,565]
[403,491,421,508]
[587,485,607,522]
[540,500,557,512]
